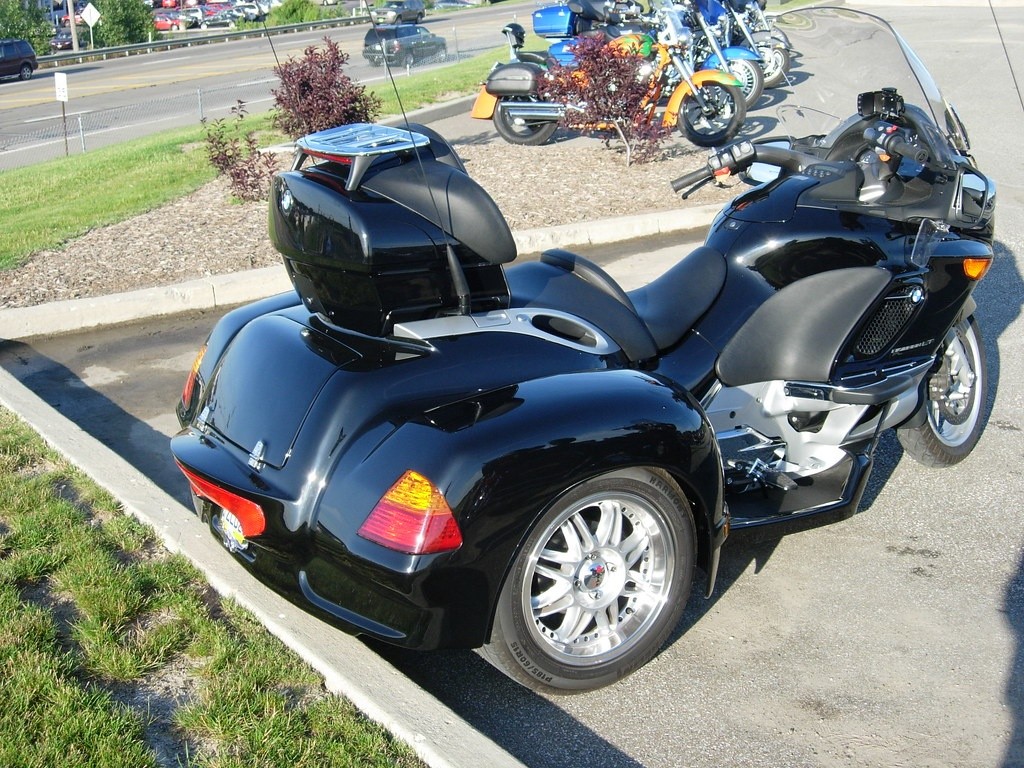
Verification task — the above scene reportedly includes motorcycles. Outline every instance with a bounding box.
[469,0,794,150]
[168,5,997,702]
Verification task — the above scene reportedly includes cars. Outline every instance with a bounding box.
[144,0,283,27]
[311,0,342,6]
[198,12,246,31]
[153,13,180,30]
[48,29,81,50]
[60,8,87,29]
[433,0,473,9]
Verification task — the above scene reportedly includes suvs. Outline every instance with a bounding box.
[363,22,448,70]
[0,39,38,82]
[371,0,426,26]
